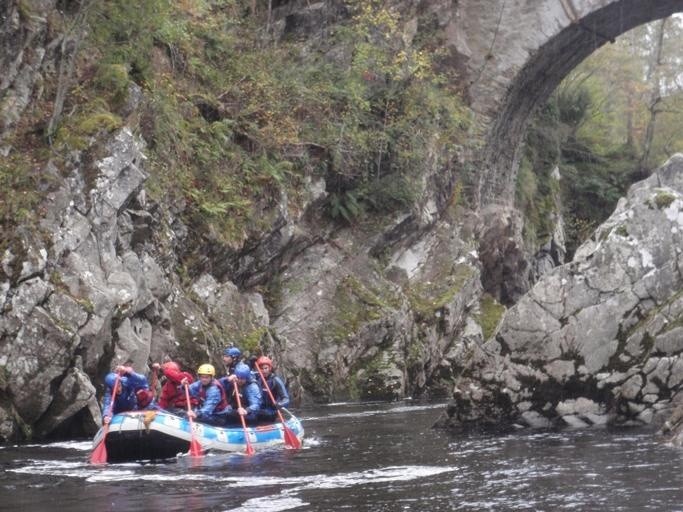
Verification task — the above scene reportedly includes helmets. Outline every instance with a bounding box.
[222,347,241,358]
[104,372,123,390]
[197,363,216,377]
[235,364,251,380]
[256,355,273,373]
[162,361,179,372]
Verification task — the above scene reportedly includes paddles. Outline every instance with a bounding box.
[229,379,254,453]
[182,382,206,457]
[254,361,302,447]
[90,369,123,463]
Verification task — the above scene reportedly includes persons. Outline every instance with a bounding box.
[102,365,155,425]
[219,364,263,428]
[152,361,200,414]
[222,346,241,375]
[180,364,229,426]
[247,356,290,422]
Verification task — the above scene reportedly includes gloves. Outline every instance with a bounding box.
[247,355,258,365]
[272,403,280,410]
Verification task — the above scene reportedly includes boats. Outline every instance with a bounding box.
[90,411,306,464]
